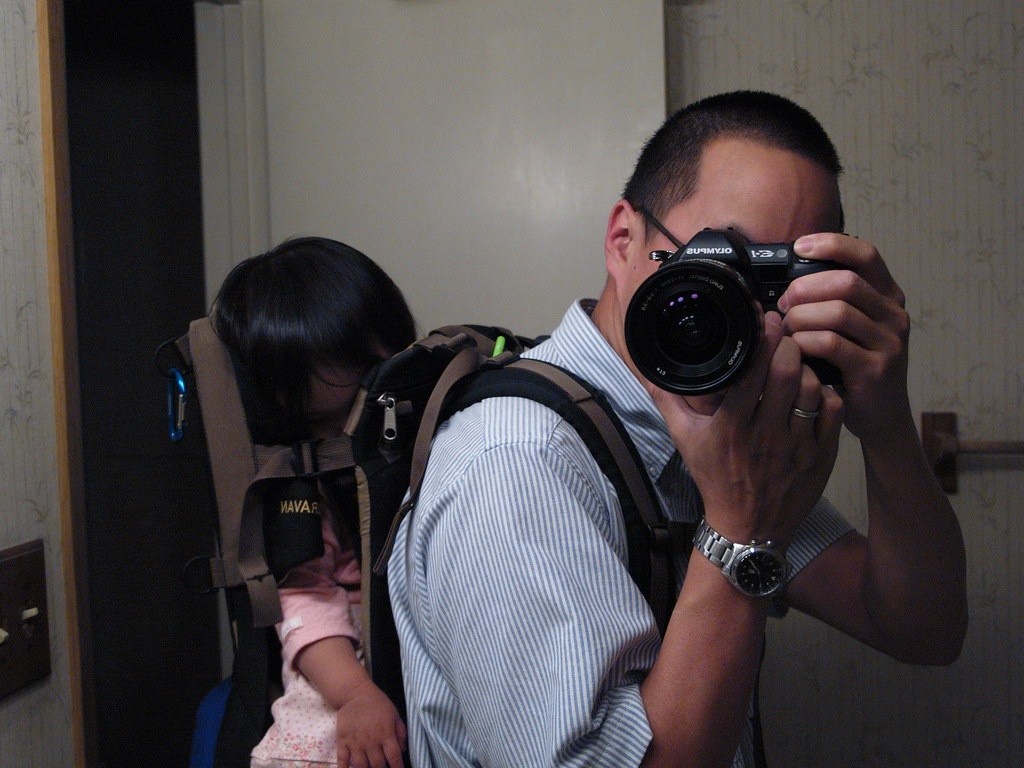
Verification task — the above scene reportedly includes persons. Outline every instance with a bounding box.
[207,235,420,768]
[386,88,970,767]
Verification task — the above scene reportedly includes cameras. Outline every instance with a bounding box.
[624,226,850,397]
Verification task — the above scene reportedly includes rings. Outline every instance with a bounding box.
[790,405,821,421]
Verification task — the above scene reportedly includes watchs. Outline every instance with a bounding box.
[691,517,794,600]
